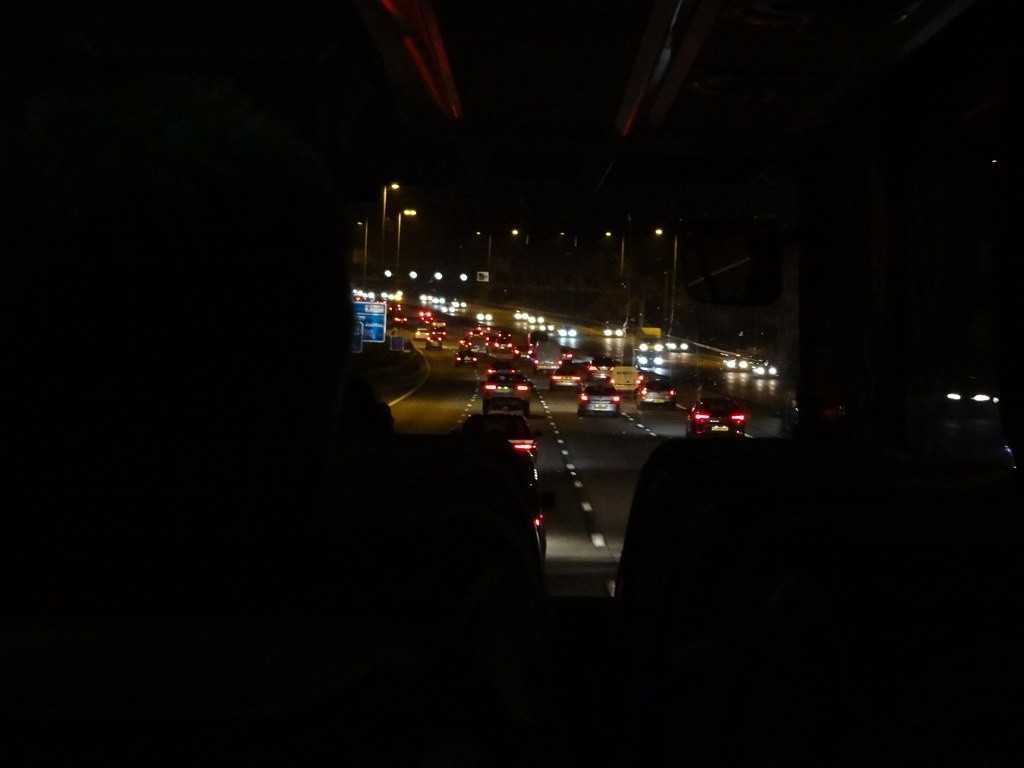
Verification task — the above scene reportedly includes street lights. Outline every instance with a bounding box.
[476,231,492,263]
[511,229,530,244]
[380,182,400,257]
[357,218,368,290]
[605,231,625,285]
[656,227,679,335]
[560,231,578,247]
[395,207,418,280]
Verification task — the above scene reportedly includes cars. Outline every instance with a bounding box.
[721,352,780,380]
[577,383,622,417]
[480,370,534,418]
[460,411,544,474]
[685,398,748,440]
[388,299,691,396]
[635,376,690,411]
